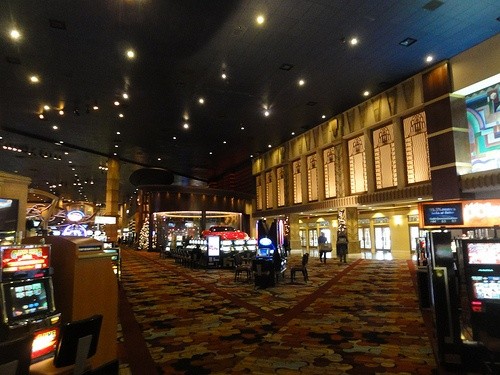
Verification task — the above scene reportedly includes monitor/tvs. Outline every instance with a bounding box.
[1,276,56,329]
[184,245,257,252]
[418,201,464,228]
[472,276,500,300]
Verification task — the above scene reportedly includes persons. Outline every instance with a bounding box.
[317,232,329,263]
[336,227,349,263]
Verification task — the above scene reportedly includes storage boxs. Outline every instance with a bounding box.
[318,243,332,253]
[338,238,346,244]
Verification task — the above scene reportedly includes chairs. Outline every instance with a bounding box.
[170,248,256,284]
[290,253,309,284]
[29,314,103,375]
